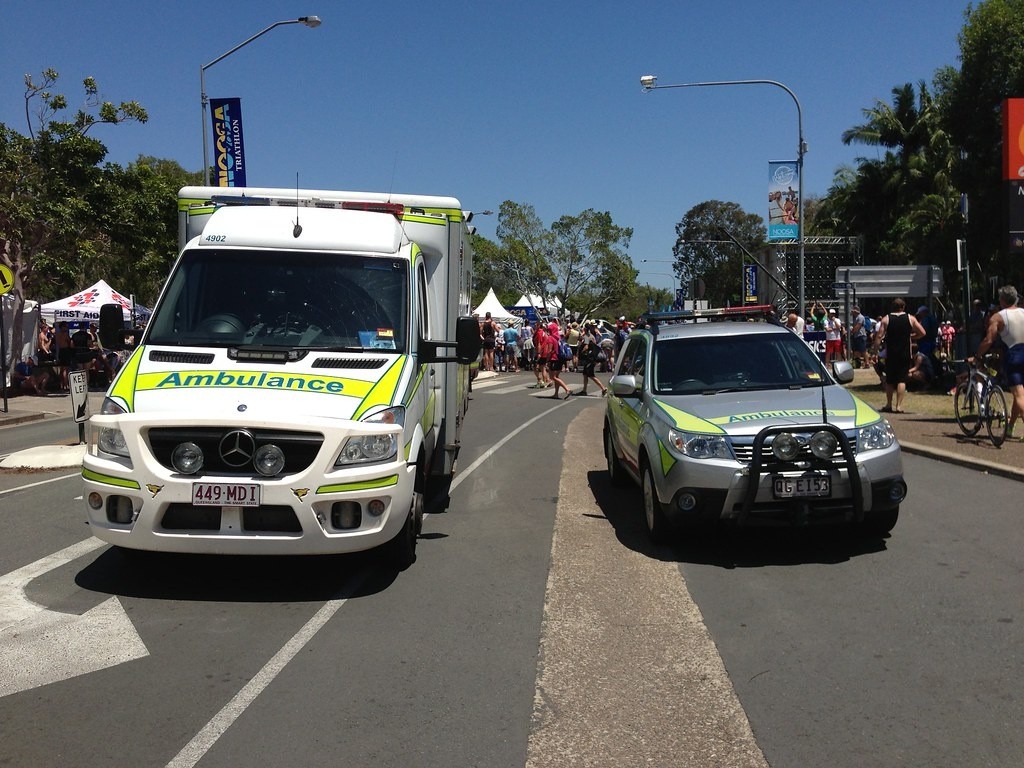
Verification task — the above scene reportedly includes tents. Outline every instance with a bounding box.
[0,278,153,389]
[471,286,570,337]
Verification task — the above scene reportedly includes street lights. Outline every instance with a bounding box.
[678,240,745,307]
[640,260,692,298]
[638,272,676,306]
[201,13,323,186]
[642,76,805,325]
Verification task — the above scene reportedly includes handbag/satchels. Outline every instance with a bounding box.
[584,341,601,362]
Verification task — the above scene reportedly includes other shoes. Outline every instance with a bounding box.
[1002,421,1015,436]
[863,364,871,369]
[572,368,577,372]
[602,388,609,397]
[576,391,588,396]
[510,366,515,369]
[563,389,573,400]
[516,370,520,372]
[560,368,564,372]
[565,368,569,372]
[878,407,892,412]
[550,394,559,399]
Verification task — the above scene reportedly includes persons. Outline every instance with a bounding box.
[778,286,1024,440]
[471,312,643,399]
[14,318,147,397]
[769,187,799,224]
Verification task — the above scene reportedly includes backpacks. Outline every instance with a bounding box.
[552,334,573,362]
[862,316,872,330]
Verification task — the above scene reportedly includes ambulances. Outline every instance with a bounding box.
[81,186,476,570]
[602,305,908,538]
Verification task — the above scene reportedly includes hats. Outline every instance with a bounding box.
[946,320,951,324]
[915,305,929,315]
[941,322,946,324]
[30,355,38,366]
[851,306,860,313]
[805,317,813,324]
[829,308,836,313]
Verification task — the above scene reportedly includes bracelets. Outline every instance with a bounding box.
[538,353,540,354]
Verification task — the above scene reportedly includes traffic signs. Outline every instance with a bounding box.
[831,282,853,289]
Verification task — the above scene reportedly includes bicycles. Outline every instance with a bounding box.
[944,355,1007,447]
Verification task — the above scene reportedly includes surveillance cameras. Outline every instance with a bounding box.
[640,75,653,85]
